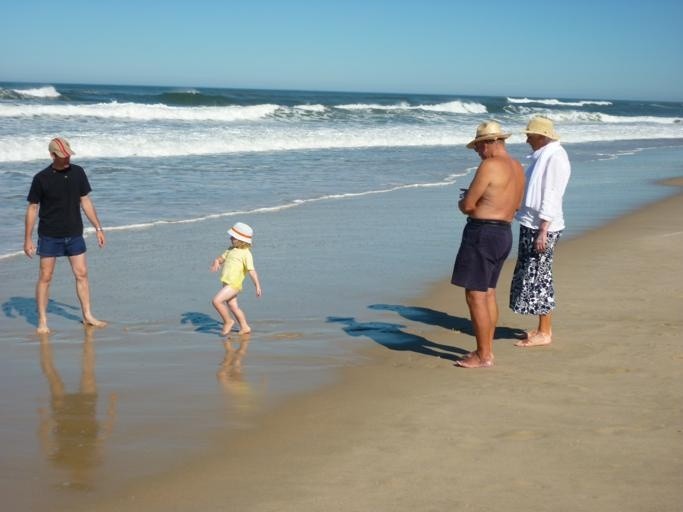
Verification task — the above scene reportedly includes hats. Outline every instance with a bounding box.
[518,114,560,141]
[226,222,255,245]
[48,137,76,159]
[466,121,513,149]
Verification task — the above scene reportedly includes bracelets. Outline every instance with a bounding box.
[96,227,102,231]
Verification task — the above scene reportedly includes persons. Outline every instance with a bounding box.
[217,337,264,411]
[24,138,107,333]
[451,121,526,369]
[38,326,117,472]
[211,222,261,336]
[508,118,571,347]
